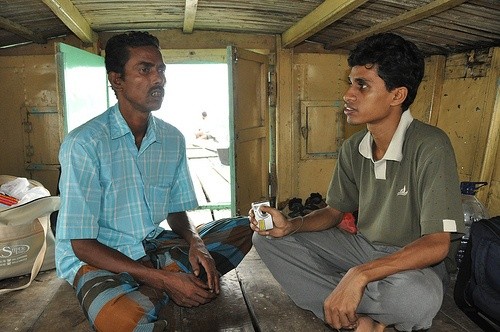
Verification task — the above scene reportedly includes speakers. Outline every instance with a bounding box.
[454,216,500,332]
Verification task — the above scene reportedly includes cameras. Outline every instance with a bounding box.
[251,200,274,232]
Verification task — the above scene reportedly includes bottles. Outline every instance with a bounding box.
[450,182,485,279]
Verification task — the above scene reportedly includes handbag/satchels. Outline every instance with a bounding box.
[454,216,500,332]
[0,175,69,293]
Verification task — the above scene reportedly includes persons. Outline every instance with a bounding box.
[196,111,214,141]
[55,31,254,332]
[249,33,466,332]
